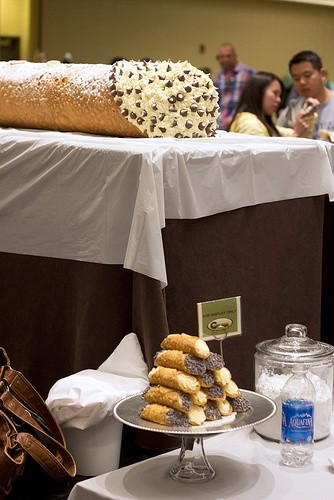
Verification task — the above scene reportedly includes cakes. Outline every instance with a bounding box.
[0,58,221,138]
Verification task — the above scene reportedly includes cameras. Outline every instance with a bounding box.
[302,104,316,118]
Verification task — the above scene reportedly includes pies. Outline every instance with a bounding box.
[141,332,252,429]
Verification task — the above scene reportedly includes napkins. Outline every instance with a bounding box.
[44,332,149,432]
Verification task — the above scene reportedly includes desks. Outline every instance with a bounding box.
[67,423,334,500]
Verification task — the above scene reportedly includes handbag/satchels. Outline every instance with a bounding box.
[0,347,78,497]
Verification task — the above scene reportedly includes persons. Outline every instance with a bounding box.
[212,42,257,132]
[285,50,334,144]
[228,71,311,138]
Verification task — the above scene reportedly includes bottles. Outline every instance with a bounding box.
[253,322,334,442]
[280,365,316,468]
